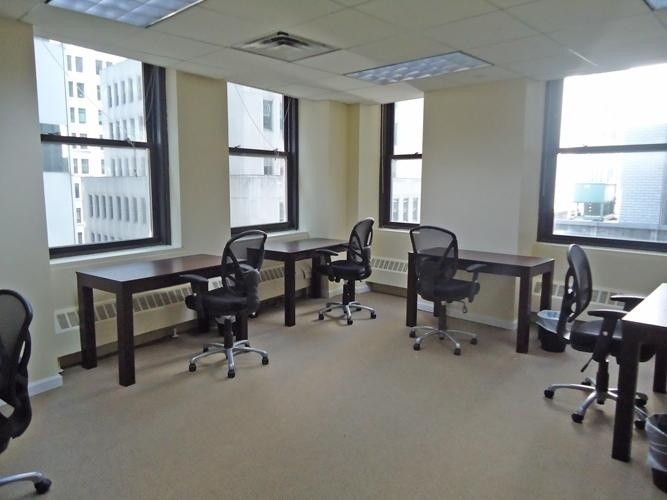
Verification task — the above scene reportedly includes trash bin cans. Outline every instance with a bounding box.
[535,310,570,355]
[212,313,242,338]
[642,410,667,494]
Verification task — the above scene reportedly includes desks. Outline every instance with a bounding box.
[72,253,252,386]
[402,248,553,354]
[239,236,362,326]
[601,281,667,466]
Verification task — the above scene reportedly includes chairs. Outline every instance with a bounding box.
[184,225,269,377]
[402,226,480,359]
[0,288,53,496]
[540,243,650,432]
[320,216,378,324]
